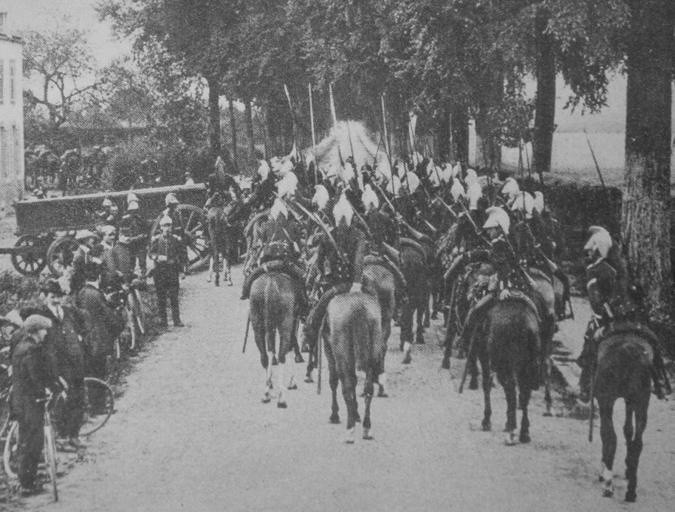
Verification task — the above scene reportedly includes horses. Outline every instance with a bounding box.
[204,183,573,447]
[579,250,658,505]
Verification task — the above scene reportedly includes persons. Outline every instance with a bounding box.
[6,193,185,496]
[581,228,669,398]
[206,149,573,338]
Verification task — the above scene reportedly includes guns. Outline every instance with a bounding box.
[273,168,559,289]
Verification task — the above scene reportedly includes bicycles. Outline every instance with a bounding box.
[36,374,114,500]
[0,360,26,482]
[103,280,146,364]
